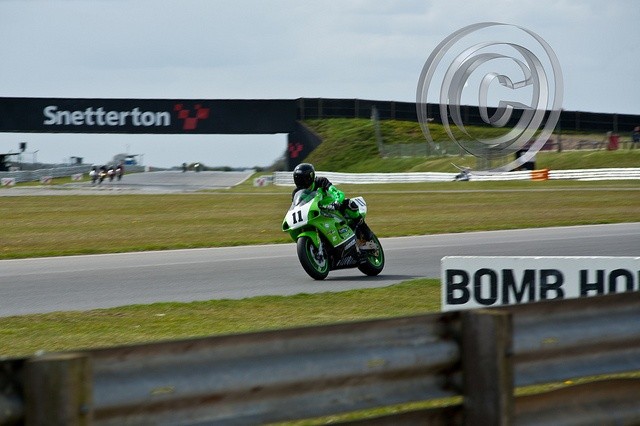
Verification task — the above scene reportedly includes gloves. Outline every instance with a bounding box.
[330,202,340,209]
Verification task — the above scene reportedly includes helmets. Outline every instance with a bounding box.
[293,163,315,187]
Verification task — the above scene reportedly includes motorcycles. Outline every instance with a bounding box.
[108,169,115,181]
[98,171,107,182]
[281,188,385,280]
[116,168,122,180]
[89,171,97,183]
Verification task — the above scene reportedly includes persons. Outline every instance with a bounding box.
[292,163,360,220]
